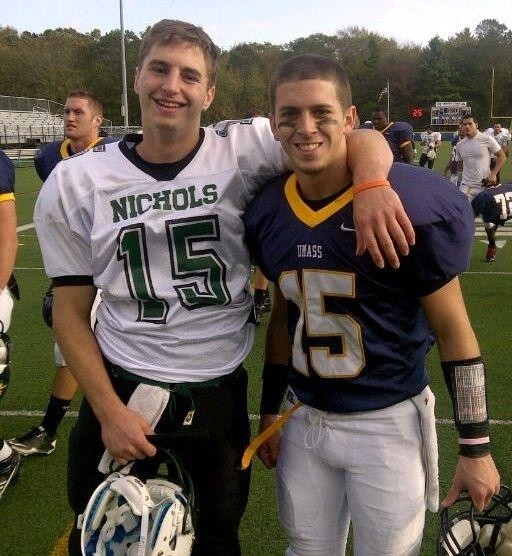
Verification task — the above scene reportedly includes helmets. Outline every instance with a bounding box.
[437,484,512,555]
[69,441,197,556]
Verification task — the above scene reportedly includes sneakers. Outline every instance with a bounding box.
[253,295,272,322]
[12,427,58,456]
[487,244,497,261]
[0,451,22,497]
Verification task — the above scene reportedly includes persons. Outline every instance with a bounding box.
[455,114,508,262]
[253,267,271,314]
[31,19,408,556]
[0,131,21,509]
[474,119,479,128]
[419,124,442,169]
[444,122,465,185]
[356,106,415,165]
[4,90,121,458]
[241,54,501,556]
[484,122,512,183]
